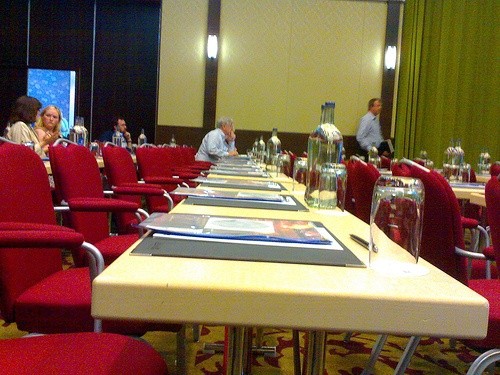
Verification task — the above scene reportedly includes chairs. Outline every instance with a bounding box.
[282,148,500,352]
[0,142,215,375]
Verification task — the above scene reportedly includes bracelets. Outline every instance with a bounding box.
[127,140,132,142]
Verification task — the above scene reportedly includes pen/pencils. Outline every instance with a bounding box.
[350,234,378,252]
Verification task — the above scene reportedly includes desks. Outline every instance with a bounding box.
[447,180,485,199]
[91,153,488,375]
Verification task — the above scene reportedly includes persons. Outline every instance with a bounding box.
[356,98,391,164]
[194,117,239,163]
[98,116,133,147]
[2,96,59,157]
[33,105,67,156]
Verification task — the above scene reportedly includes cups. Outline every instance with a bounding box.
[274,154,348,211]
[462,162,475,182]
[367,175,425,268]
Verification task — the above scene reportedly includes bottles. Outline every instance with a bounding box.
[253,128,281,173]
[72,115,88,147]
[441,138,491,182]
[369,142,379,169]
[113,128,146,148]
[304,100,347,206]
[170,135,176,147]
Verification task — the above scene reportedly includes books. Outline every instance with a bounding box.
[379,139,394,157]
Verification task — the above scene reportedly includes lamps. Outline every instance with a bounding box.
[207,32,218,67]
[386,45,397,73]
